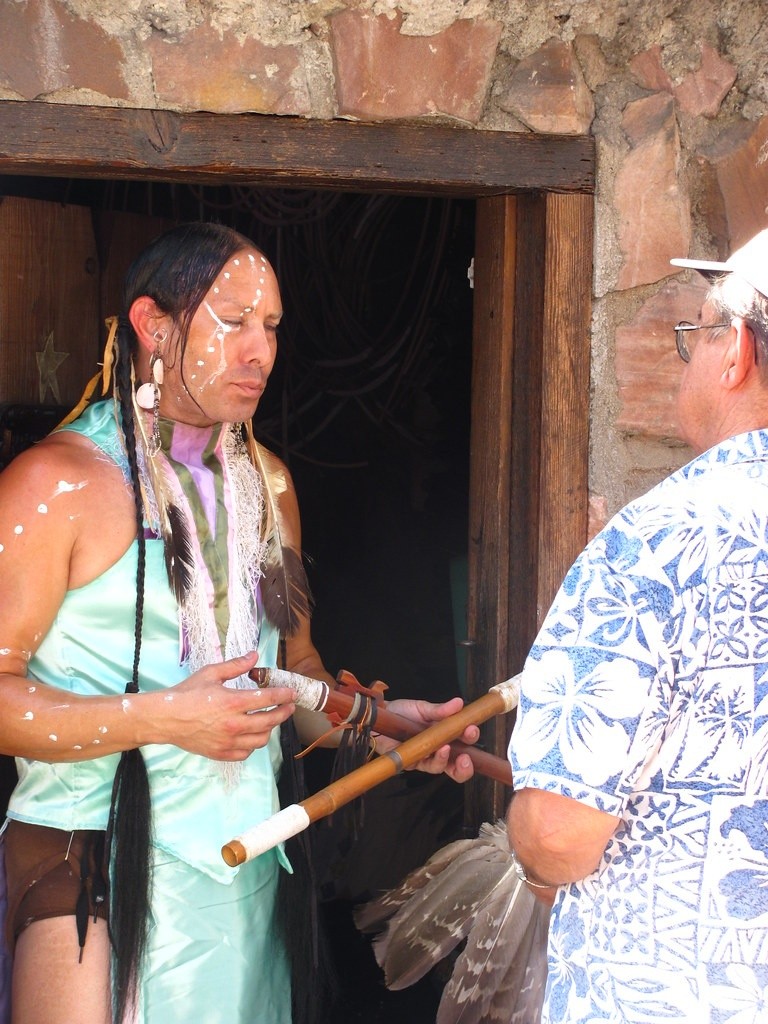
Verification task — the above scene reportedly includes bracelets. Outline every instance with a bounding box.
[511,854,551,891]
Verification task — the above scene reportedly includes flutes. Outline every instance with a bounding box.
[222,668,523,866]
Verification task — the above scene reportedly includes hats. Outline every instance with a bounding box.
[670,227,768,302]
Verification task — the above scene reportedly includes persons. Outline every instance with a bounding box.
[499,226,768,1024]
[0,219,480,1024]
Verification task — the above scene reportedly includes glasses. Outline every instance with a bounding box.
[672,321,759,370]
